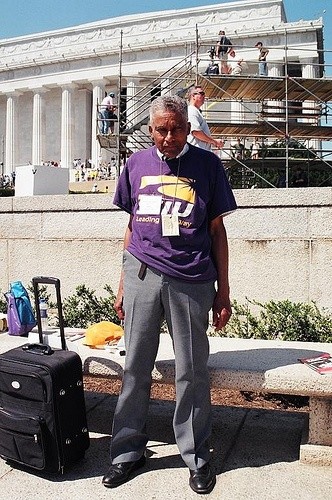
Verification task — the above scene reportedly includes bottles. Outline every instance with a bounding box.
[39,299,48,331]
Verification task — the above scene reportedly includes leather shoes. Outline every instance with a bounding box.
[188,461,216,494]
[101,448,146,489]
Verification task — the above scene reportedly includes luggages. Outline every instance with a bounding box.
[0,276,91,476]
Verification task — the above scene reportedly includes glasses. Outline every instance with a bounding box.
[193,92,205,96]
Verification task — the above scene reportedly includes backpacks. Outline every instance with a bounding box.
[4,281,37,336]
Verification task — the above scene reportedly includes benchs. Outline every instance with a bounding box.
[0,313,332,446]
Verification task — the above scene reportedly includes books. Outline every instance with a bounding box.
[297,353,332,375]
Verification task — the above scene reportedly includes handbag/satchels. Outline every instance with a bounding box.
[82,321,124,346]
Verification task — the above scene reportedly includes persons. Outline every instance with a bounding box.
[92,183,99,194]
[105,186,109,193]
[204,58,220,75]
[184,86,224,152]
[102,96,238,495]
[98,92,118,134]
[27,161,32,165]
[251,137,261,159]
[228,51,243,75]
[215,30,232,74]
[73,155,118,181]
[0,172,16,189]
[231,139,244,160]
[40,160,62,167]
[255,41,269,76]
